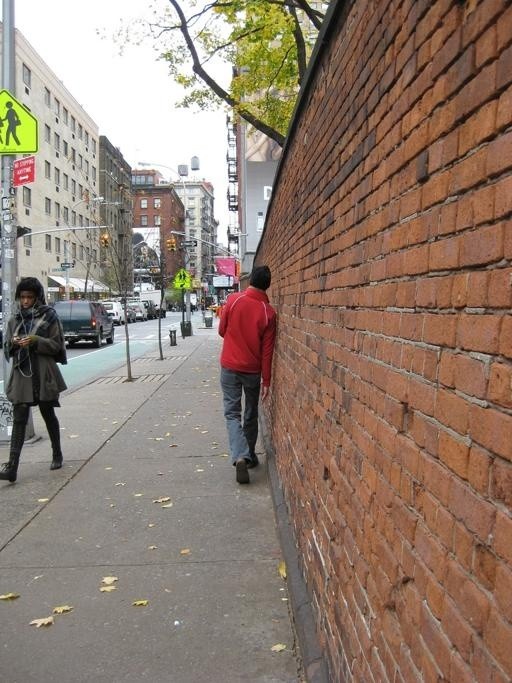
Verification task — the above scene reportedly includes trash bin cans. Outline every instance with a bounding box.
[180,321,191,336]
[204,316,213,327]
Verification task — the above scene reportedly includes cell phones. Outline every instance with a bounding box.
[16,338,21,340]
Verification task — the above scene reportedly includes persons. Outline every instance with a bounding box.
[217,265,276,483]
[0,276,68,483]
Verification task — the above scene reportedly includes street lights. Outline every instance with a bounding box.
[132,241,148,297]
[139,162,194,336]
[65,196,105,300]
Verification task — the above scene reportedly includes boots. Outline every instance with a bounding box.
[44,417,63,470]
[0,422,26,483]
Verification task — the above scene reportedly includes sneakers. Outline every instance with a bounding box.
[235,456,259,484]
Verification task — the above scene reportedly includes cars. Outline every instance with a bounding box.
[99,289,167,327]
[172,291,220,313]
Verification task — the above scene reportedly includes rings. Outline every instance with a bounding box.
[22,338,24,340]
[23,341,25,342]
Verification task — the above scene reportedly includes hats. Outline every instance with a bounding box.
[249,265,272,289]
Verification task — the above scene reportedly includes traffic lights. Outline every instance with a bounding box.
[101,234,105,248]
[105,235,110,248]
[167,240,172,252]
[171,239,178,252]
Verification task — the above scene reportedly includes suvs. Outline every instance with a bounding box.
[53,299,116,350]
